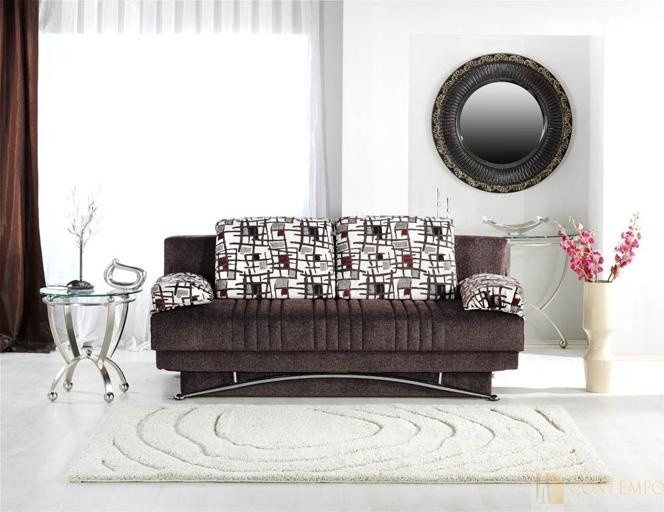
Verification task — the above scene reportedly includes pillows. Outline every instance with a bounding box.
[214,216,337,300]
[334,214,458,301]
[151,272,214,314]
[460,273,525,320]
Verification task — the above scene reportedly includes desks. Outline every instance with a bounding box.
[504,234,579,349]
[39,284,143,403]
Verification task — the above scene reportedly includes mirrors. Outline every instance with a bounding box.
[432,52,573,194]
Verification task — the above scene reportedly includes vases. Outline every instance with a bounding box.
[582,280,612,393]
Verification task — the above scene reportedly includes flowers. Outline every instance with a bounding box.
[554,214,641,282]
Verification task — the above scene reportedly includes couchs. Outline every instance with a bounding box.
[149,232,524,399]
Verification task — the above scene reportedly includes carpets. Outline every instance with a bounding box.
[65,401,609,485]
